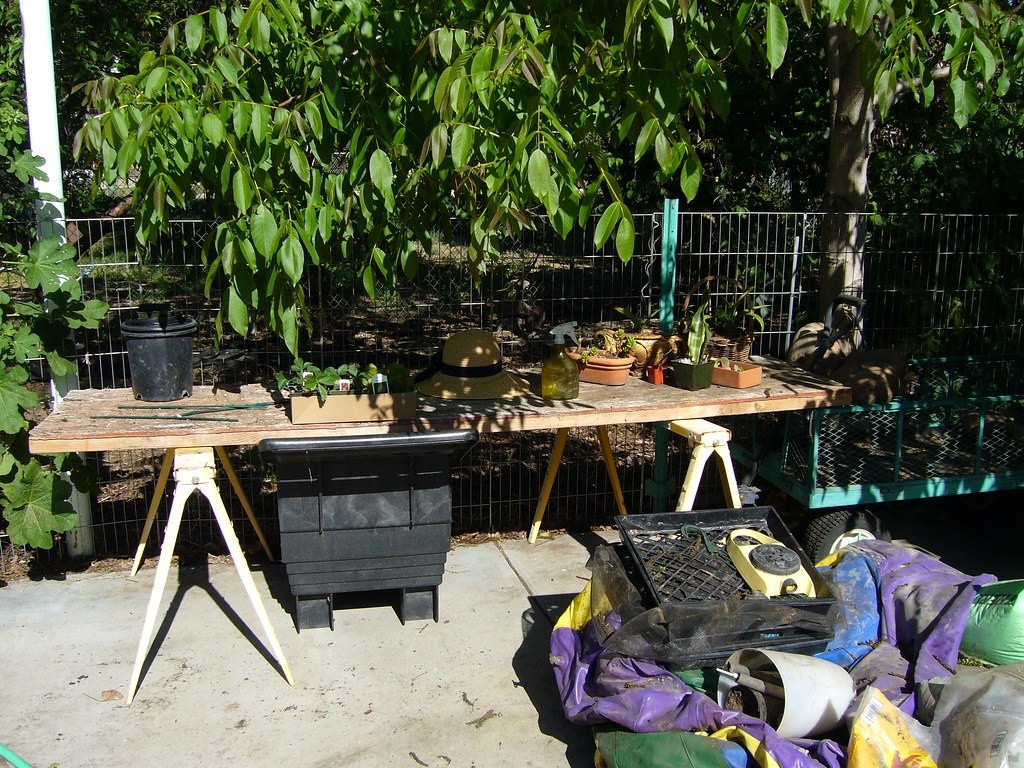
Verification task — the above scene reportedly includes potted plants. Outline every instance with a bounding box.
[565,326,636,386]
[647,284,772,391]
[275,358,419,424]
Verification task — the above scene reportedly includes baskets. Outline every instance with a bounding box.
[678,275,755,363]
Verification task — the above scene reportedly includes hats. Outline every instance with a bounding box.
[416,329,530,399]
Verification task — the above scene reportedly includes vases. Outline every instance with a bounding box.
[718,648,857,740]
[120,314,198,402]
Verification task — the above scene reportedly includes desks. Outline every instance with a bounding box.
[28,355,852,705]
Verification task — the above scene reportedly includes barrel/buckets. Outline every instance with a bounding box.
[120,316,197,402]
[717,647,857,739]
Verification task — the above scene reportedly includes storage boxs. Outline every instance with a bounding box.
[257,429,480,633]
[601,504,840,670]
